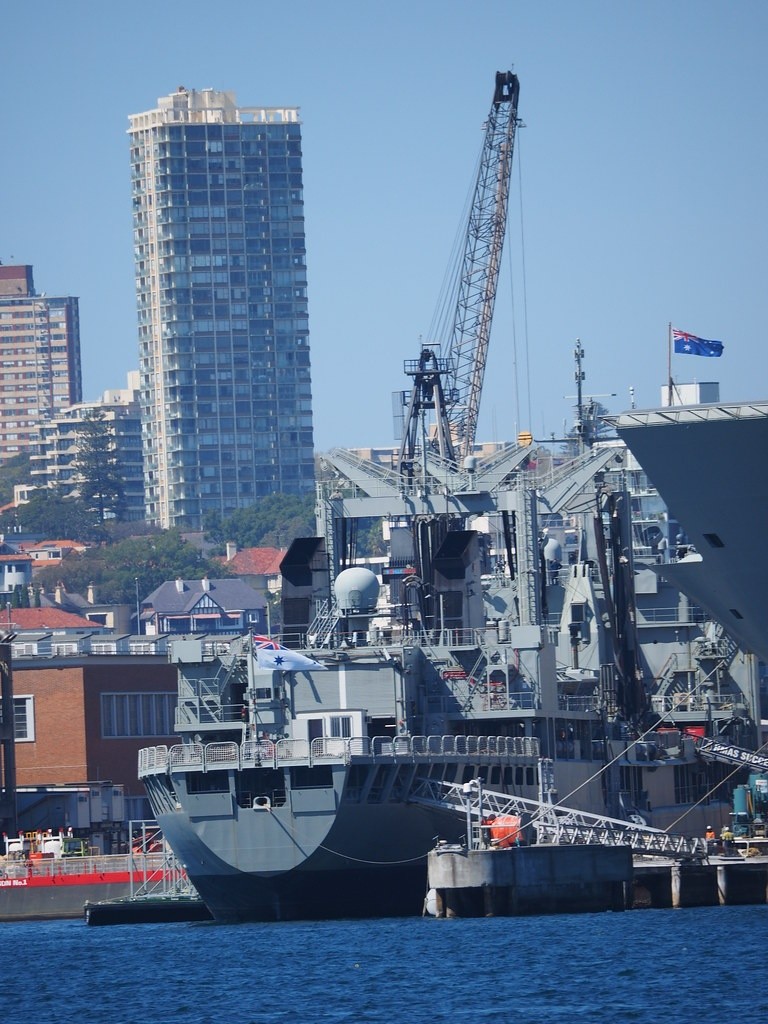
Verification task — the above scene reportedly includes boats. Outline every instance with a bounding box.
[133,347,768,917]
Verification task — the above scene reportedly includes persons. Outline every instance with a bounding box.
[721,826,735,857]
[706,826,718,856]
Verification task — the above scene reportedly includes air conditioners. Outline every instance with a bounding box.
[675,694,692,705]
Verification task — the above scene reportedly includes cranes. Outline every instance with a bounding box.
[397,68,525,494]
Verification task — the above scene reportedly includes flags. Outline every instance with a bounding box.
[253,632,329,672]
[672,327,725,358]
